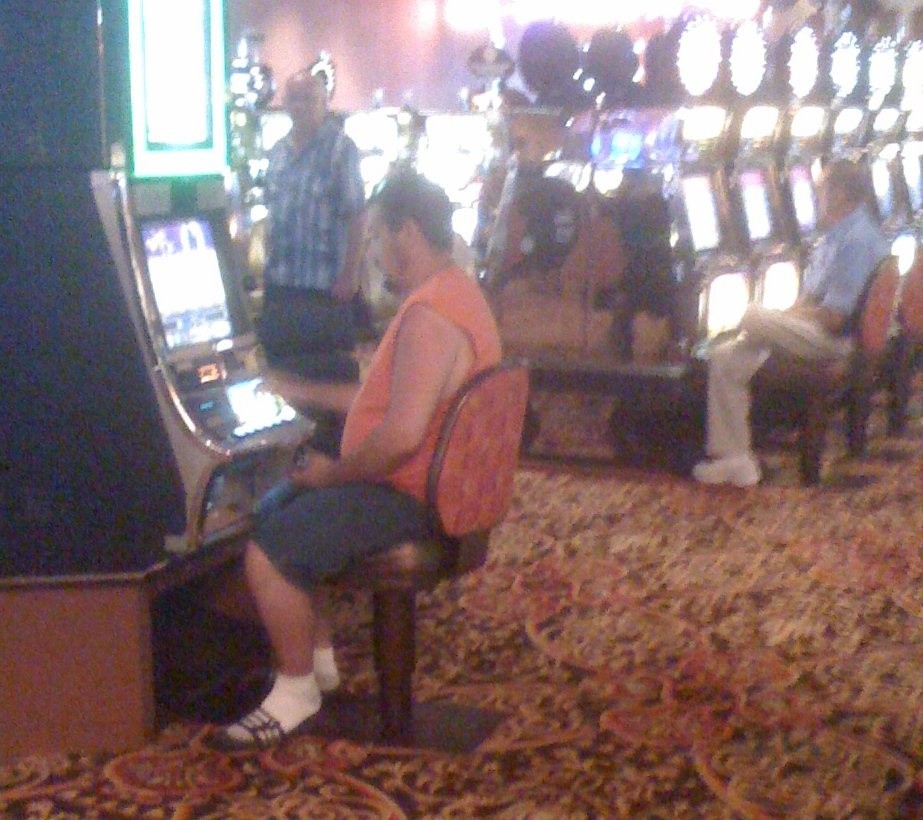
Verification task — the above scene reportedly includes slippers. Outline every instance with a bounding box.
[234,667,341,699]
[217,706,324,748]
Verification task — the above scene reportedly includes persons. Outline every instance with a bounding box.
[689,157,895,488]
[258,70,369,461]
[206,170,505,754]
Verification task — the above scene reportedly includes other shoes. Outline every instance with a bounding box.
[691,458,763,487]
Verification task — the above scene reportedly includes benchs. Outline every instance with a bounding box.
[517,356,691,469]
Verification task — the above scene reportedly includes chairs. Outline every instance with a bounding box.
[747,261,900,488]
[844,249,922,430]
[317,360,529,741]
